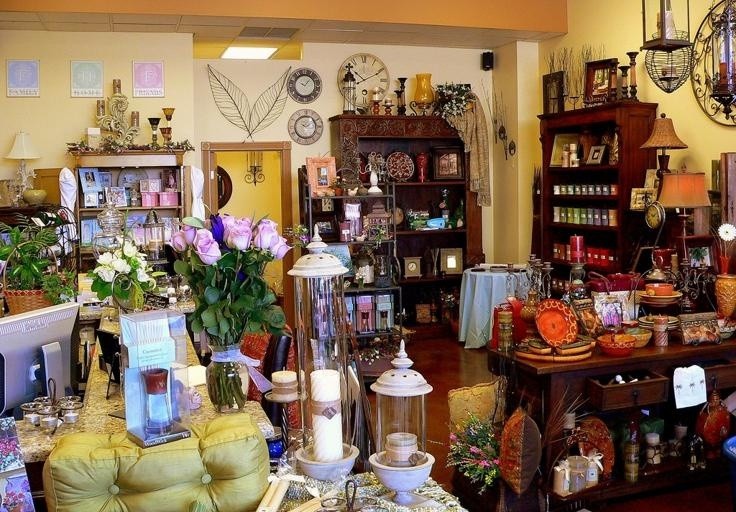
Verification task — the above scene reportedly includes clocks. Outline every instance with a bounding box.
[286,52,392,146]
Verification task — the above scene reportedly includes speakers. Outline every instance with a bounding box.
[483,52,494,71]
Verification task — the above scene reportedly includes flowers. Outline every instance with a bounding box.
[84,204,172,315]
[169,196,300,412]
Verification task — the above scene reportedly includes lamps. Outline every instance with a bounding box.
[243,147,268,190]
[1,126,44,206]
[338,61,443,116]
[94,78,177,151]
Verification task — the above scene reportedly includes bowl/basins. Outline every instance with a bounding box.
[622,327,653,349]
[645,283,674,295]
[596,334,637,358]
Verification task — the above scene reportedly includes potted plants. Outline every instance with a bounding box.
[0,206,82,315]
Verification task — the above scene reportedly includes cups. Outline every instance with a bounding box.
[22,395,81,430]
[506,254,552,270]
[651,248,677,271]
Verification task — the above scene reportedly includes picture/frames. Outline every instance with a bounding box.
[582,57,618,108]
[542,70,564,113]
[79,167,162,211]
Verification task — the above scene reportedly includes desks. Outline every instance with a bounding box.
[0,265,466,511]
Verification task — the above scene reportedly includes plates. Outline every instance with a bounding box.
[534,297,580,349]
[637,316,681,332]
[638,291,683,303]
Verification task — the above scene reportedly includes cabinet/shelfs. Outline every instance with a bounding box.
[299,98,736,512]
[70,147,191,273]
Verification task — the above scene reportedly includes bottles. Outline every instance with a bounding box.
[563,143,581,170]
[155,273,191,307]
[553,460,567,495]
[624,443,640,485]
[266,426,286,461]
[430,294,441,324]
[652,317,670,347]
[442,208,450,221]
[672,253,679,273]
[568,455,588,495]
[140,369,173,438]
[415,151,429,182]
[497,311,513,352]
[643,433,664,468]
[347,310,388,331]
[583,447,601,487]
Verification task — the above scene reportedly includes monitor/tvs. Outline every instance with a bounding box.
[0,302,80,421]
